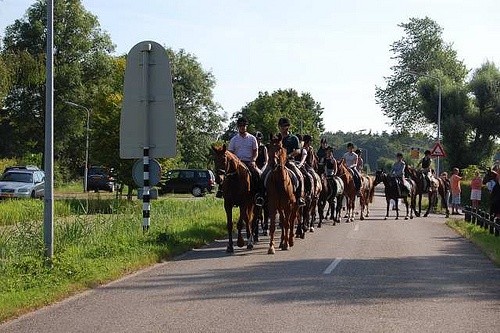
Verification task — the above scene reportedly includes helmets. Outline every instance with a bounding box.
[355,149,362,157]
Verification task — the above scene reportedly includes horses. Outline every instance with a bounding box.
[209,144,450,255]
[483,166,500,223]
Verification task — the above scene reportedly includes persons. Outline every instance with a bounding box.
[355,149,363,173]
[483,167,497,185]
[303,135,319,200]
[471,170,482,208]
[317,138,333,161]
[493,165,500,185]
[390,153,411,196]
[252,131,269,206]
[416,149,432,191]
[276,117,306,206]
[441,172,451,217]
[294,134,313,200]
[342,143,362,195]
[450,167,465,215]
[216,117,261,197]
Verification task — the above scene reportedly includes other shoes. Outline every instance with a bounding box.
[331,197,336,203]
[297,197,306,206]
[357,190,363,195]
[255,192,265,206]
[216,183,223,198]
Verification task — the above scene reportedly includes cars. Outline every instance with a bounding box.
[0,169,45,201]
[2,165,40,175]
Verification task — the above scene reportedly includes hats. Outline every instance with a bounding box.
[424,150,431,154]
[236,117,249,127]
[253,131,262,140]
[347,143,354,148]
[303,134,314,141]
[397,152,404,157]
[296,134,302,140]
[278,118,291,126]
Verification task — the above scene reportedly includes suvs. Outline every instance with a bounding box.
[83,166,114,193]
[158,168,216,197]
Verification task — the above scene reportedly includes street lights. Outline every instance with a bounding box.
[350,129,366,142]
[64,101,89,193]
[408,71,442,177]
